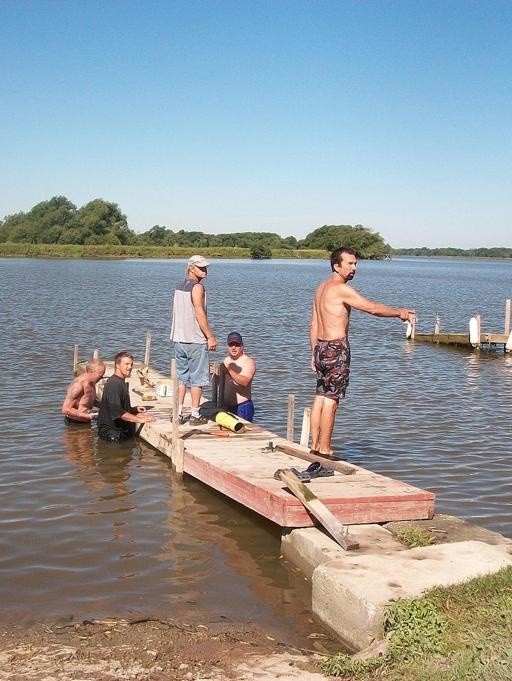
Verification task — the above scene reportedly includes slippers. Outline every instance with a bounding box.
[301,461,334,477]
[274,467,311,483]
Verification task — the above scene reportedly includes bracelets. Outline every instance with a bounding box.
[207,336,214,340]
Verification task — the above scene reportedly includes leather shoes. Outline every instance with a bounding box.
[310,449,319,455]
[318,450,348,460]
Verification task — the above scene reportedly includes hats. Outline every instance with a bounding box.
[188,254,212,269]
[227,331,243,343]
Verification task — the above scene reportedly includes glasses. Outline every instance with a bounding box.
[228,340,242,347]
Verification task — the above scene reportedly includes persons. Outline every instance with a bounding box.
[168,254,218,425]
[96,352,154,445]
[309,246,415,464]
[61,358,107,429]
[208,331,257,422]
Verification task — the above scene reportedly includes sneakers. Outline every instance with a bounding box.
[171,414,188,423]
[189,414,208,424]
